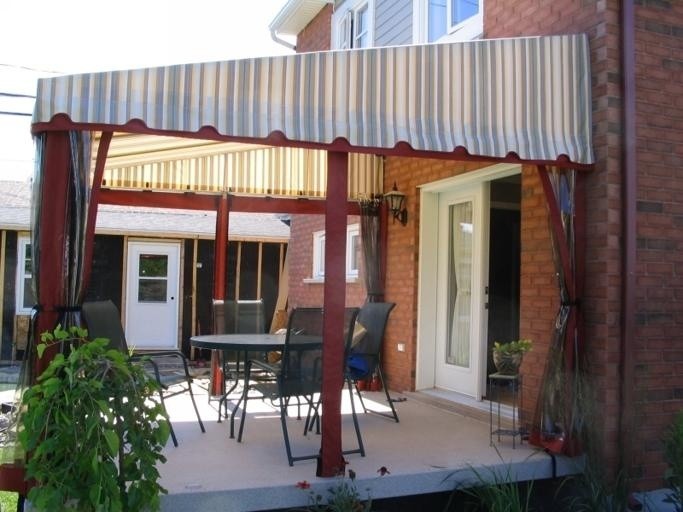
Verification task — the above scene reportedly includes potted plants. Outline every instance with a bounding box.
[491,338,534,377]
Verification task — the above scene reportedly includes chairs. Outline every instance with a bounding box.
[237,308,366,466]
[303,302,399,434]
[212,298,323,438]
[83,299,207,447]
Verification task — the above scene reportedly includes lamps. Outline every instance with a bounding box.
[383,180,407,227]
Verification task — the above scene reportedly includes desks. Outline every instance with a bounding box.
[189,332,335,441]
[488,372,527,450]
